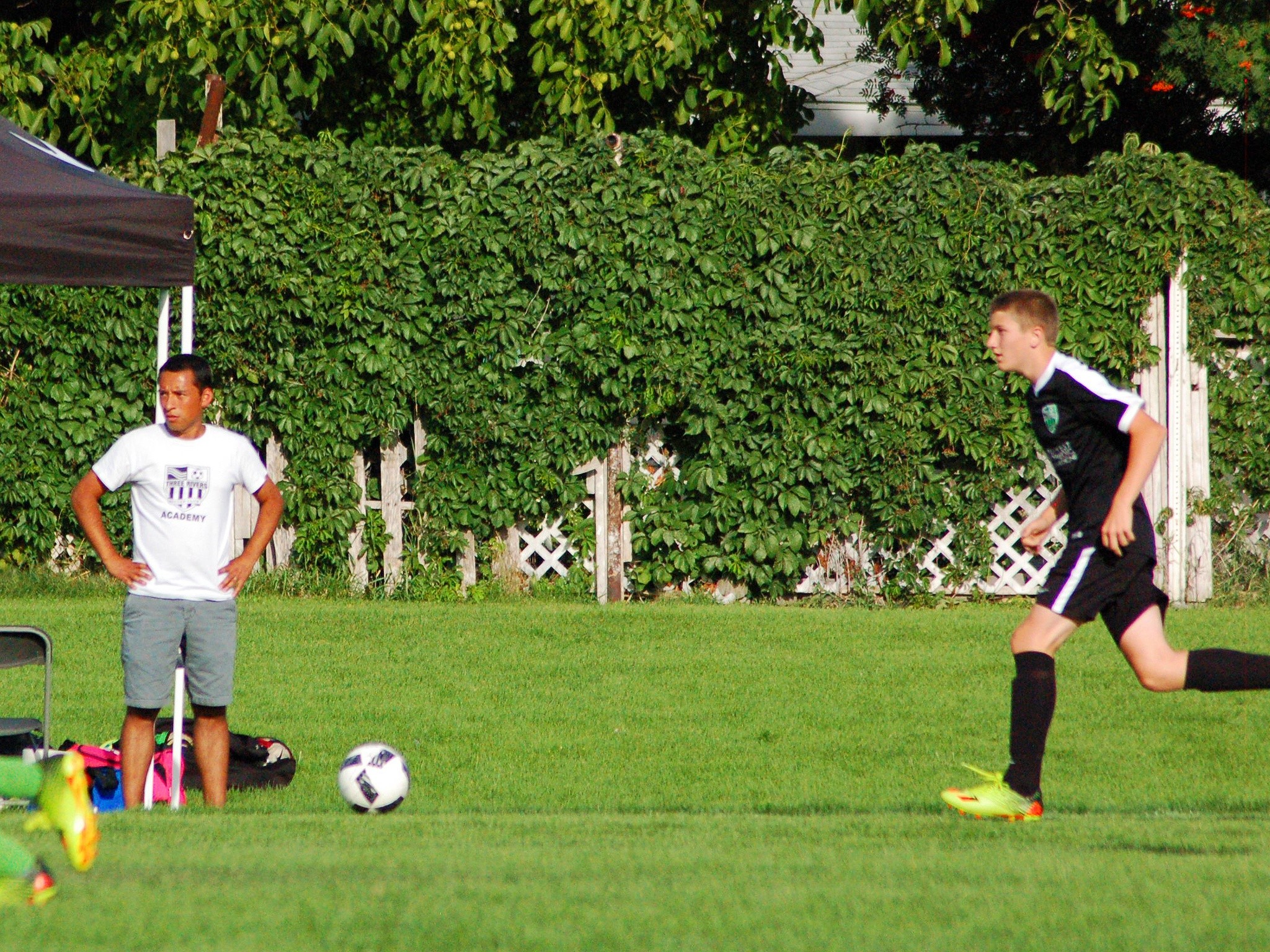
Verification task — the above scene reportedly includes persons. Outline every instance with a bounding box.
[72,355,283,811]
[940,289,1270,824]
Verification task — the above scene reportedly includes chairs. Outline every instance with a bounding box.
[0,621,55,762]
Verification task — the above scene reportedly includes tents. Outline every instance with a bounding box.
[0,118,197,810]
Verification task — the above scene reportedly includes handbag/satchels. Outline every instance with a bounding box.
[59,739,186,811]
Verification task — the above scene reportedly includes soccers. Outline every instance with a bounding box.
[338,742,411,815]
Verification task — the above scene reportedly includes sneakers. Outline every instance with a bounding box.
[34,752,101,872]
[0,859,58,907]
[940,761,1044,820]
